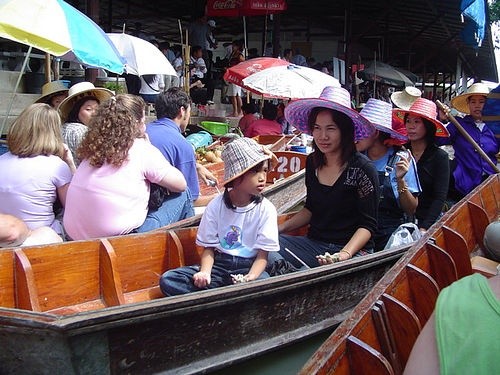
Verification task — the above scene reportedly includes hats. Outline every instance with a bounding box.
[391,87,422,110]
[221,138,279,187]
[33,81,69,103]
[57,82,114,121]
[283,86,376,144]
[392,98,450,138]
[359,98,409,140]
[451,83,489,113]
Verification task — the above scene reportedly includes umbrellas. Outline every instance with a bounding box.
[1,0,125,77]
[357,61,416,90]
[105,23,178,78]
[224,57,350,102]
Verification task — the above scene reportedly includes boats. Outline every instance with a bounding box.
[150,151,308,228]
[298,173,500,375]
[200,134,286,165]
[0,209,416,375]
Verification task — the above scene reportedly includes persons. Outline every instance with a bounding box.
[441,82,499,199]
[223,38,246,116]
[396,263,499,374]
[355,98,422,254]
[280,87,377,275]
[140,75,164,103]
[0,101,79,249]
[133,86,199,235]
[61,92,195,240]
[393,97,450,239]
[34,79,69,105]
[179,19,221,86]
[58,81,115,168]
[161,137,280,298]
